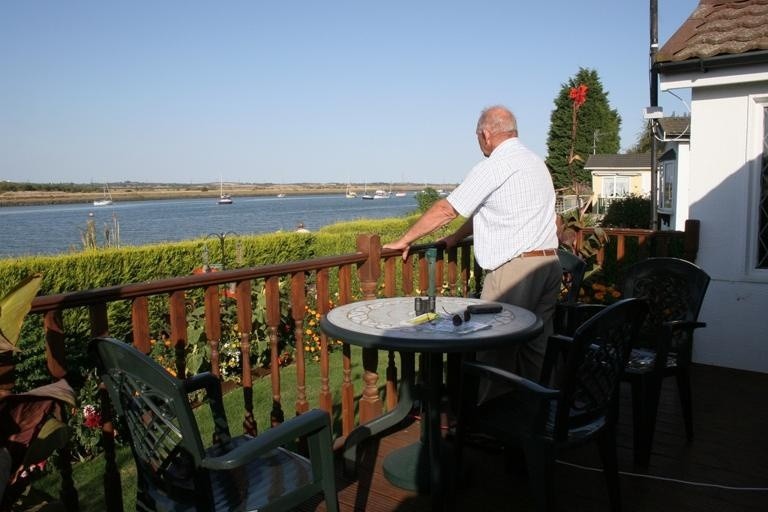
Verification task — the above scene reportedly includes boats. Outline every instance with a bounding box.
[217,193,233,204]
[276,193,286,198]
[93,199,112,207]
[345,189,425,199]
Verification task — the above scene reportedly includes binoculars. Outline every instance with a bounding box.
[415,296,435,316]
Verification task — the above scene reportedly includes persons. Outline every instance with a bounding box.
[381,102,567,459]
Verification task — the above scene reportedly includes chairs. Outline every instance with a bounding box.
[85,333,342,512]
[553,250,586,339]
[459,296,650,510]
[574,255,710,462]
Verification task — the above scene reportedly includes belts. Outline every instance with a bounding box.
[518,249,558,258]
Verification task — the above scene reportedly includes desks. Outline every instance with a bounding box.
[320,294,545,493]
